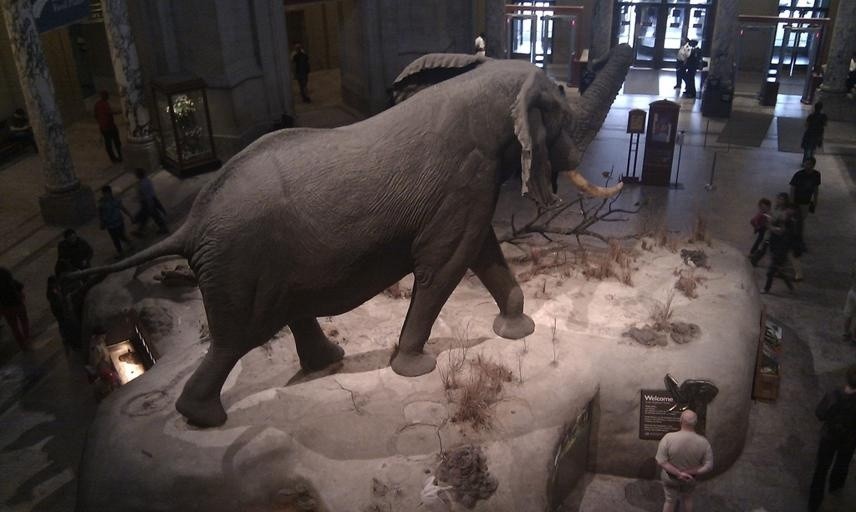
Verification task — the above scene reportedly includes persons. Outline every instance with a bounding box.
[93,88,124,164]
[54,256,84,311]
[5,107,39,154]
[807,362,856,511]
[654,408,714,511]
[56,228,93,270]
[747,155,822,295]
[800,102,828,168]
[673,37,693,90]
[683,47,700,98]
[474,32,488,57]
[0,266,31,353]
[97,185,134,257]
[134,167,169,233]
[45,275,73,358]
[289,44,311,104]
[842,284,856,344]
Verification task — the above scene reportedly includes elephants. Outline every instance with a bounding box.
[61,42,637,430]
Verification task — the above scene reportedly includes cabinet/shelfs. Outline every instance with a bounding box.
[153,77,221,177]
